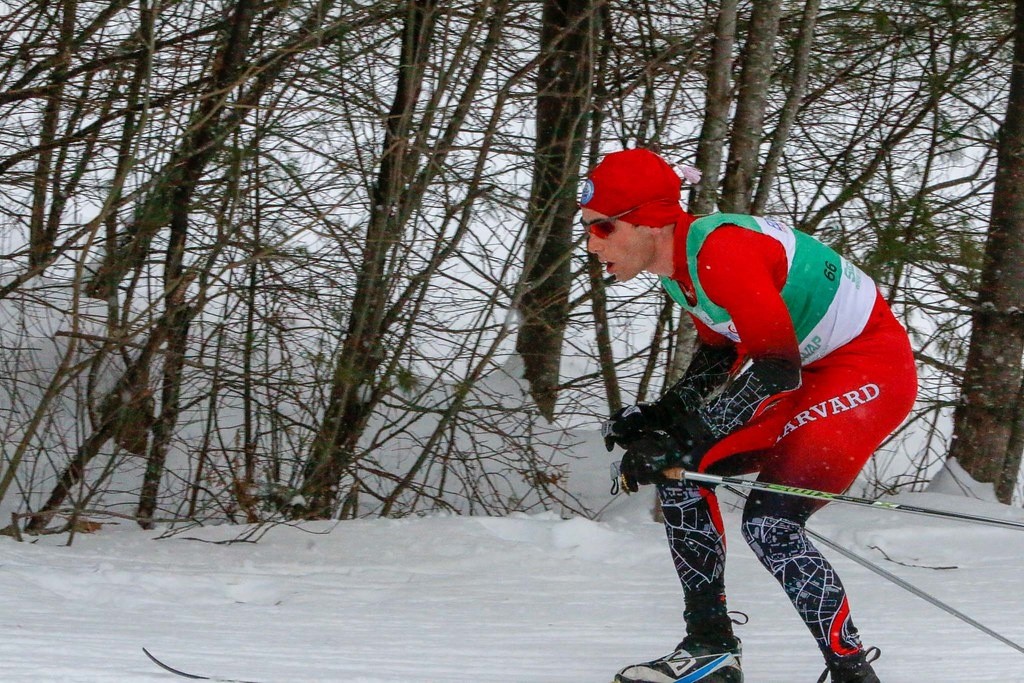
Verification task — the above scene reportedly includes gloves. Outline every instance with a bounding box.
[620,407,720,495]
[602,390,686,447]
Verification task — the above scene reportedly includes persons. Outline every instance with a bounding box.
[581,148,917,682]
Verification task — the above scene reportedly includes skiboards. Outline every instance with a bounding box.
[141,646,260,683]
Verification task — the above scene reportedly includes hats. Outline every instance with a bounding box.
[580,148,683,228]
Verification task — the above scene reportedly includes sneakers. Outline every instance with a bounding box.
[614,611,748,683]
[817,647,882,683]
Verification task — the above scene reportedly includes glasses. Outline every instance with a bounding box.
[580,202,653,239]
